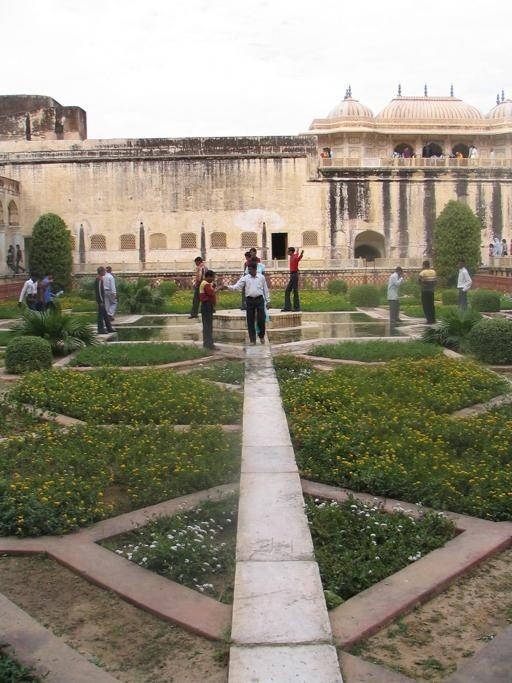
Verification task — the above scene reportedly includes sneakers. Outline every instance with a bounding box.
[188,315,198,319]
[424,320,436,325]
[203,343,219,351]
[97,328,119,335]
[279,308,302,313]
[248,337,266,346]
[388,318,405,326]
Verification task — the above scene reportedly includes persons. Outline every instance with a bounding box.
[419,261,437,324]
[15,245,25,273]
[17,271,41,311]
[95,267,119,334]
[188,257,216,319]
[386,267,404,336]
[281,247,304,312]
[392,144,478,159]
[222,248,271,346]
[102,266,118,320]
[199,270,224,350]
[456,261,473,312]
[40,272,56,310]
[489,238,512,257]
